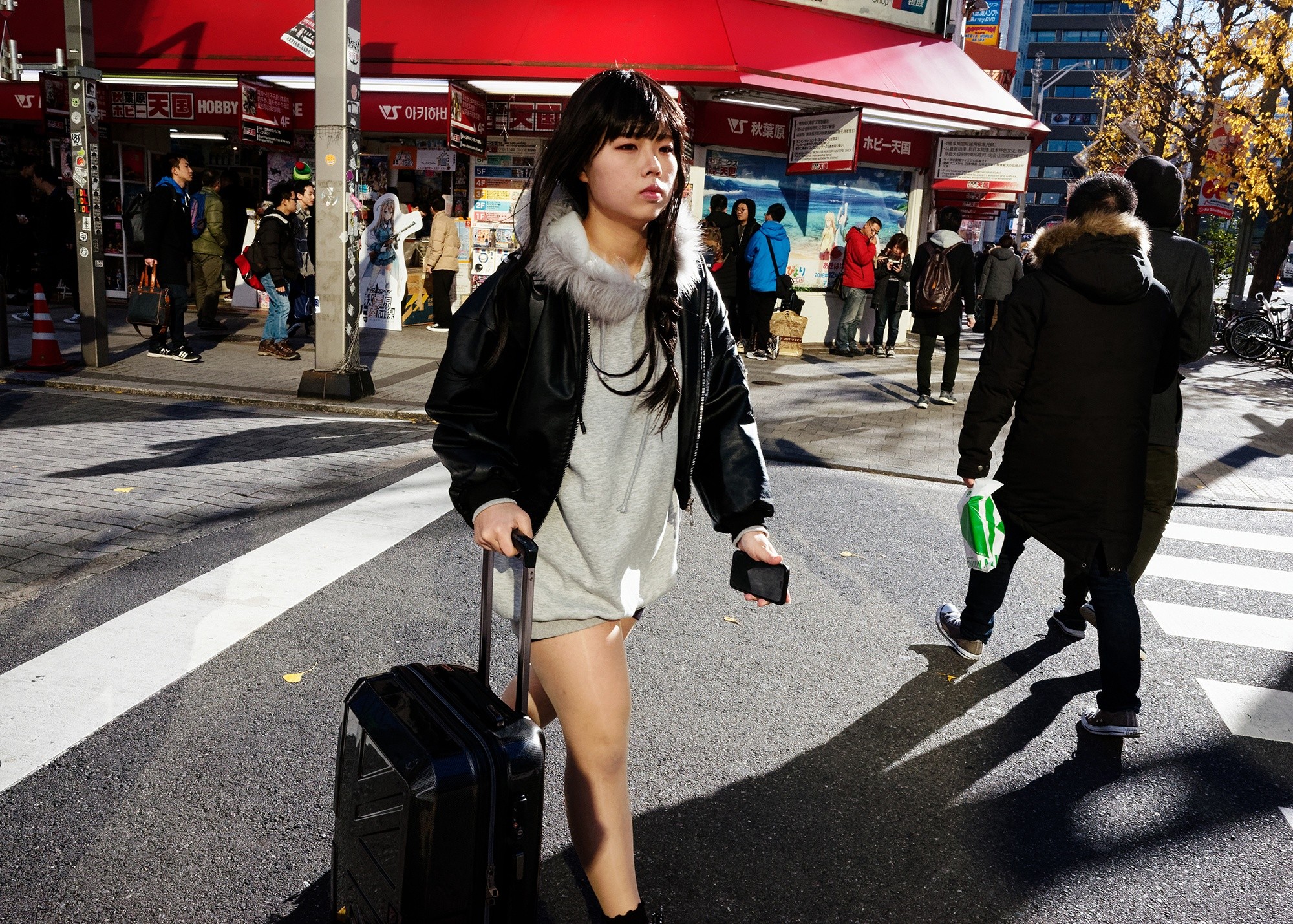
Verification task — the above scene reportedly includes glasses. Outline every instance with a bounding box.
[286,197,299,203]
[735,207,748,213]
[869,223,878,235]
[764,213,768,216]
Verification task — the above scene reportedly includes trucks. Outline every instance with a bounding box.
[1281,240,1293,281]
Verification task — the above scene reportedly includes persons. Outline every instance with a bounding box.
[743,202,792,361]
[286,161,316,337]
[245,181,302,361]
[730,198,763,354]
[188,169,228,331]
[1052,152,1216,639]
[422,64,778,924]
[907,204,976,409]
[696,193,740,343]
[834,216,883,358]
[4,153,41,308]
[142,152,203,363]
[399,198,429,239]
[422,196,461,333]
[11,161,81,325]
[934,169,1184,739]
[869,233,913,359]
[287,180,319,338]
[972,230,1031,344]
[217,170,249,302]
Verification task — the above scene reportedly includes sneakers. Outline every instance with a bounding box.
[223,289,234,301]
[63,313,80,324]
[1053,598,1148,661]
[936,603,984,661]
[147,344,202,362]
[833,343,896,358]
[737,335,781,361]
[11,312,33,322]
[913,390,958,409]
[258,323,302,360]
[426,323,451,332]
[1081,707,1141,738]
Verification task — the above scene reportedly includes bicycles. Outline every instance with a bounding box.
[1210,291,1293,376]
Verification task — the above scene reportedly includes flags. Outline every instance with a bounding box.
[1195,98,1253,219]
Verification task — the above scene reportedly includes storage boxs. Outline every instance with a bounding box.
[402,265,433,327]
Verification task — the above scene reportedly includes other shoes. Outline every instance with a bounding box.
[197,318,229,331]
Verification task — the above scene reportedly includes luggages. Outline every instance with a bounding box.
[331,528,547,924]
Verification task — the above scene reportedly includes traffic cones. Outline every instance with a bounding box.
[25,282,69,368]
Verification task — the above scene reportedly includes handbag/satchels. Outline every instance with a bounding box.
[776,273,805,316]
[245,242,271,278]
[288,285,311,321]
[125,260,171,340]
[769,310,808,358]
[956,477,1006,574]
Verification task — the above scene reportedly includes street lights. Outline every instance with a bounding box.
[1015,53,1146,251]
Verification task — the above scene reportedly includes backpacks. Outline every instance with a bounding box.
[190,190,214,240]
[914,241,967,313]
[121,182,179,254]
[701,218,731,272]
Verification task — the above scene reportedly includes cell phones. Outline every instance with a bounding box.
[877,257,889,263]
[891,262,900,273]
[728,549,790,606]
